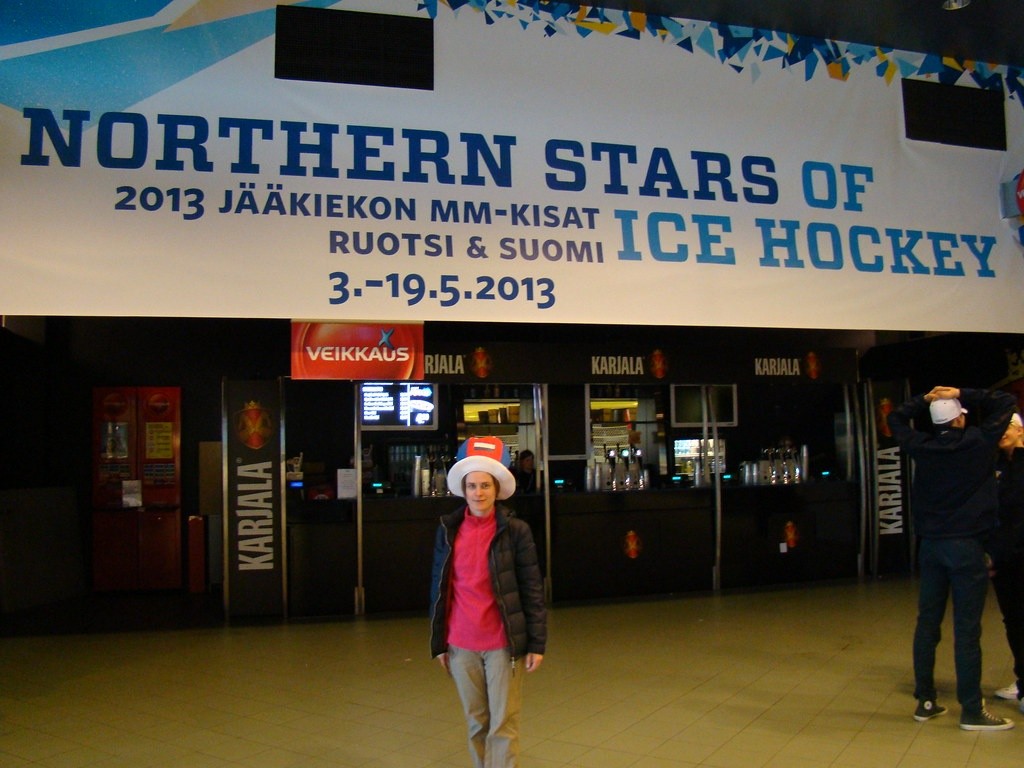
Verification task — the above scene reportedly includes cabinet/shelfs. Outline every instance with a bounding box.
[90,386,185,591]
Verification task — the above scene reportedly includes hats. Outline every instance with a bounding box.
[1010,413,1023,427]
[929,396,968,424]
[446,436,516,501]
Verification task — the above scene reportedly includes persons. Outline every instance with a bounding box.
[986,405,1024,711]
[886,386,1015,733]
[509,451,544,494]
[431,455,548,768]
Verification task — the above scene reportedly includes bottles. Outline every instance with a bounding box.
[389,444,450,462]
[431,469,437,495]
[462,384,518,398]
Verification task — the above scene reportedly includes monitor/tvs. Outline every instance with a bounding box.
[361,381,438,430]
[670,384,738,426]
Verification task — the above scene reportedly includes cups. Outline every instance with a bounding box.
[500,408,506,423]
[421,469,430,495]
[479,411,488,422]
[434,469,447,495]
[489,409,497,423]
[508,406,520,423]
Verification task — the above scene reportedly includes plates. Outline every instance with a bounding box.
[389,468,411,484]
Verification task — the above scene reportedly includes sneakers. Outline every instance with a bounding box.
[913,697,948,721]
[995,682,1019,699]
[959,697,1015,730]
